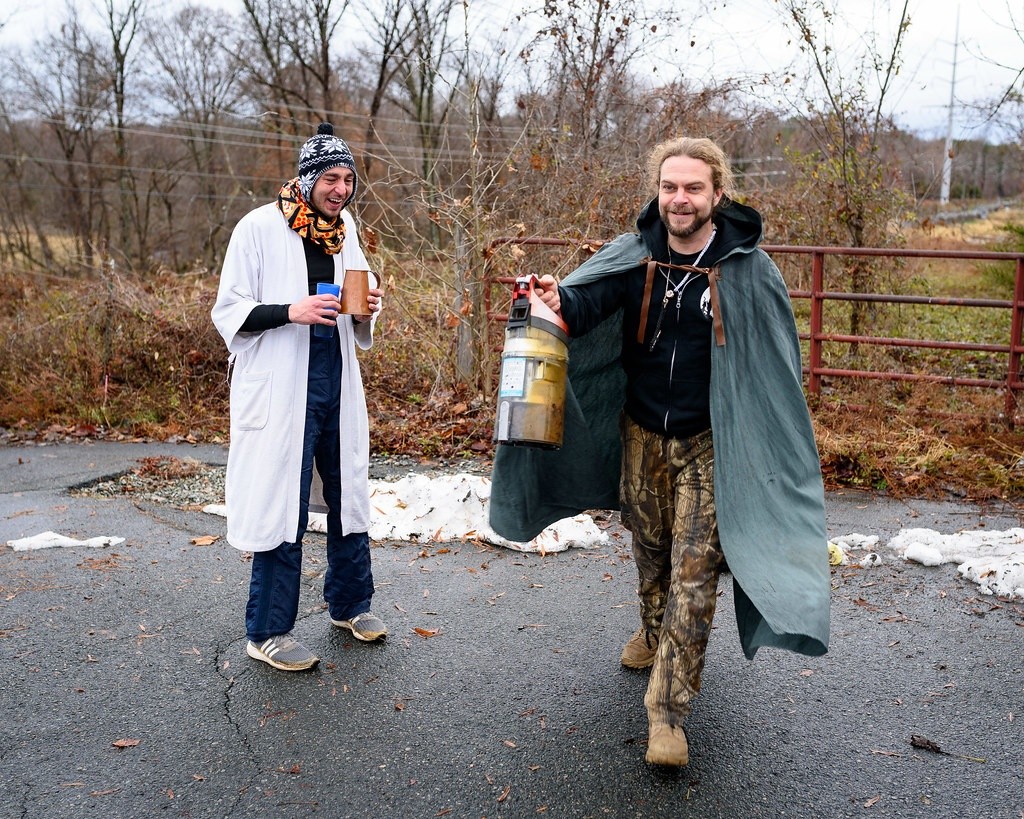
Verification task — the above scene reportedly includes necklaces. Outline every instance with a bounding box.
[646,230,719,352]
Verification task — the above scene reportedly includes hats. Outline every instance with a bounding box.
[298,122,357,207]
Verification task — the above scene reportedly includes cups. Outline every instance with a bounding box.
[338,269,381,315]
[314,283,340,338]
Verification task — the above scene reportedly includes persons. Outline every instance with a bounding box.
[488,136,831,765]
[211,122,387,672]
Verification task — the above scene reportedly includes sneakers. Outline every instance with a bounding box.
[645,722,690,766]
[621,626,657,669]
[247,632,320,671]
[330,610,387,643]
